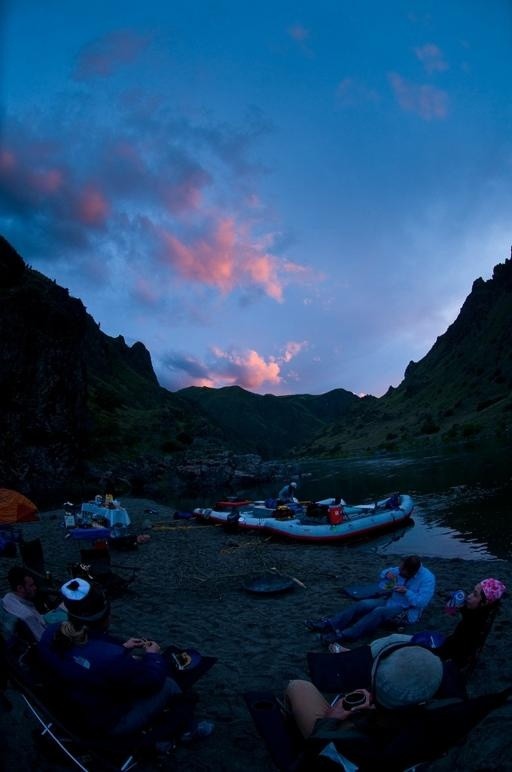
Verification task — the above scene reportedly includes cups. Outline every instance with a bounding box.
[343,692,366,711]
[114,501,121,508]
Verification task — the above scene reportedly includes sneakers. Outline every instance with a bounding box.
[303,615,343,633]
[319,634,347,649]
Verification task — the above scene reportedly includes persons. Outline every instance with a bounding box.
[0,565,77,664]
[327,577,509,710]
[301,555,438,647]
[26,577,220,750]
[276,639,475,772]
[277,481,298,504]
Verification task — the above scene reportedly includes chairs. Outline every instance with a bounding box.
[1,578,216,770]
[252,584,512,772]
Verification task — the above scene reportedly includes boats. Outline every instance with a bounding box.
[232,495,348,534]
[189,498,269,525]
[348,519,419,553]
[262,492,418,544]
[234,472,267,482]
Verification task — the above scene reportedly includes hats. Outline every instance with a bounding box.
[371,637,445,711]
[58,577,112,635]
